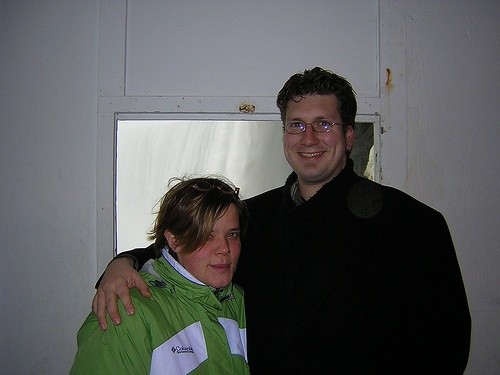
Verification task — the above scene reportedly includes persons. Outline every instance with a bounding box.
[93,67,472,375]
[70,177,249,375]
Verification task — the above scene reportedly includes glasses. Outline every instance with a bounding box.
[284,119,345,135]
[192,180,242,194]
